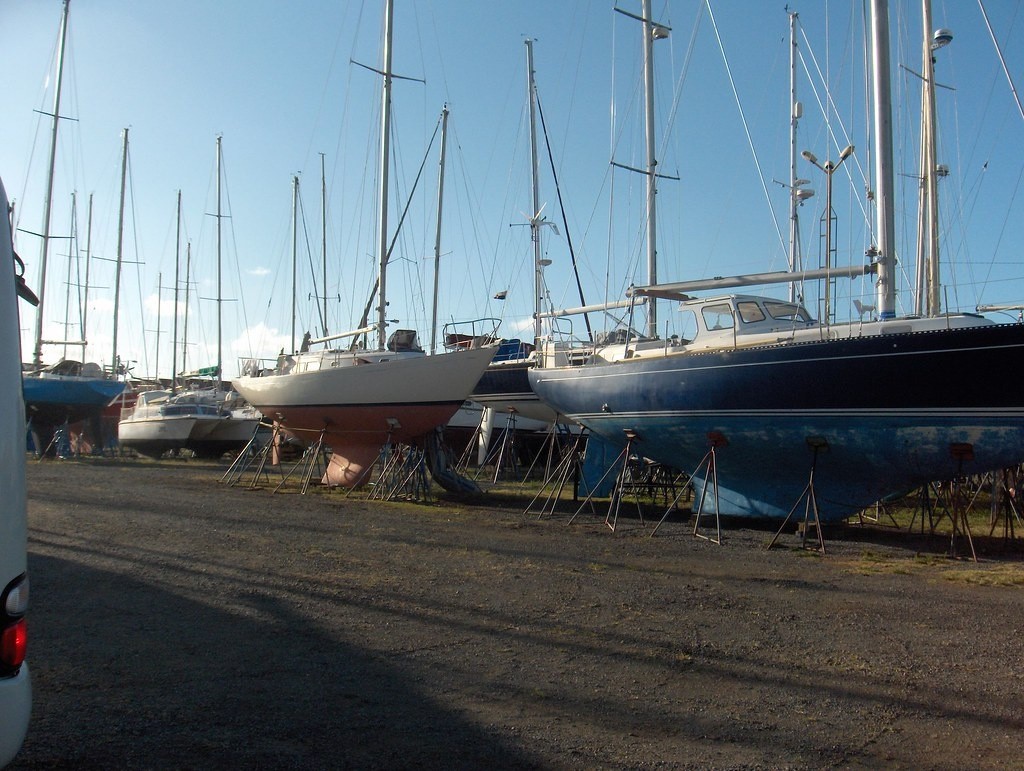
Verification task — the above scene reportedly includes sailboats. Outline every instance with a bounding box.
[3,0,1024,530]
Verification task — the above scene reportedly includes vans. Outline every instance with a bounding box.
[1,181,44,771]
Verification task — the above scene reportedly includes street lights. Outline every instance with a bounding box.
[800,144,855,326]
